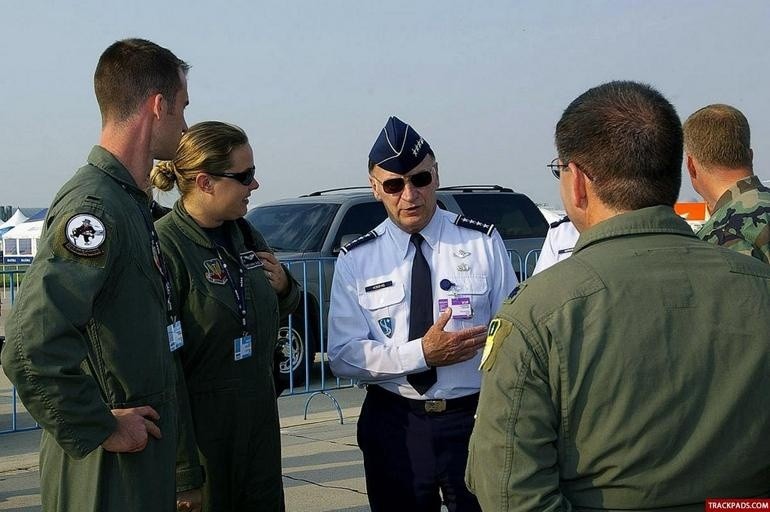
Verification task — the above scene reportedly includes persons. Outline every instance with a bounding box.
[149,121,300,512]
[1,38,190,510]
[327,116,518,511]
[682,103,769,261]
[462,81,770,511]
[531,215,580,275]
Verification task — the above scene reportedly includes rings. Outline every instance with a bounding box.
[267,271,271,278]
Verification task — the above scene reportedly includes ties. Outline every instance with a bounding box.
[407,235,437,396]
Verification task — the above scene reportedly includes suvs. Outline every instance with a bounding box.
[243,181,553,387]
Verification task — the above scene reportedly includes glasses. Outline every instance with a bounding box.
[547,158,593,182]
[207,166,255,186]
[372,165,432,194]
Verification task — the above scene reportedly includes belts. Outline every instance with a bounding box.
[367,384,479,412]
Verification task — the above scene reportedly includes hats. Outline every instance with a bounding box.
[369,116,430,175]
